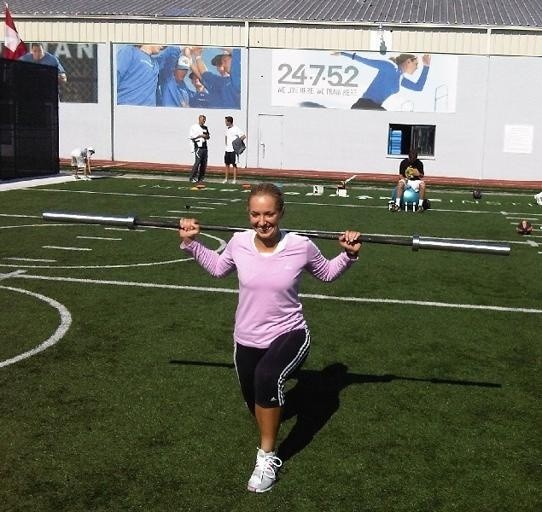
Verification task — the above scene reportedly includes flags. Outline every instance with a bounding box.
[4,7,29,60]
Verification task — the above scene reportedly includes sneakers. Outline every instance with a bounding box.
[391,204,400,212]
[74,175,82,179]
[85,176,91,179]
[245,446,280,494]
[222,179,228,184]
[232,180,237,185]
[415,206,424,213]
[189,177,209,183]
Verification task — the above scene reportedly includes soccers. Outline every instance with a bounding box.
[518,221,532,235]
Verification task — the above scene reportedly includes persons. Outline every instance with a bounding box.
[22,44,67,86]
[178,184,361,494]
[221,117,246,184]
[70,147,95,181]
[389,150,426,213]
[189,115,210,184]
[331,52,431,111]
[117,45,241,110]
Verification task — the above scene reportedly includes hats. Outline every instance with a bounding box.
[88,147,95,153]
[211,51,232,67]
[175,55,191,71]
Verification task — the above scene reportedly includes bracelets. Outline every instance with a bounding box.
[345,247,358,257]
